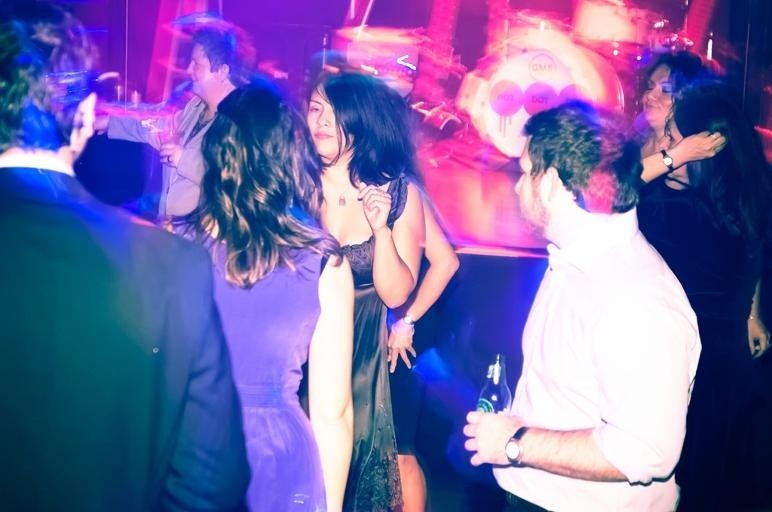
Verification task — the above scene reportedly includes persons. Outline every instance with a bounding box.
[463,100,701,512]
[616,49,771,512]
[0,0,461,512]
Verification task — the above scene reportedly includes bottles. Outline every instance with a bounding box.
[478,352,512,415]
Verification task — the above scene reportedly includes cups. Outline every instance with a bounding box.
[156,131,186,145]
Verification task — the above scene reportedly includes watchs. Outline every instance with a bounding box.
[505,427,529,467]
